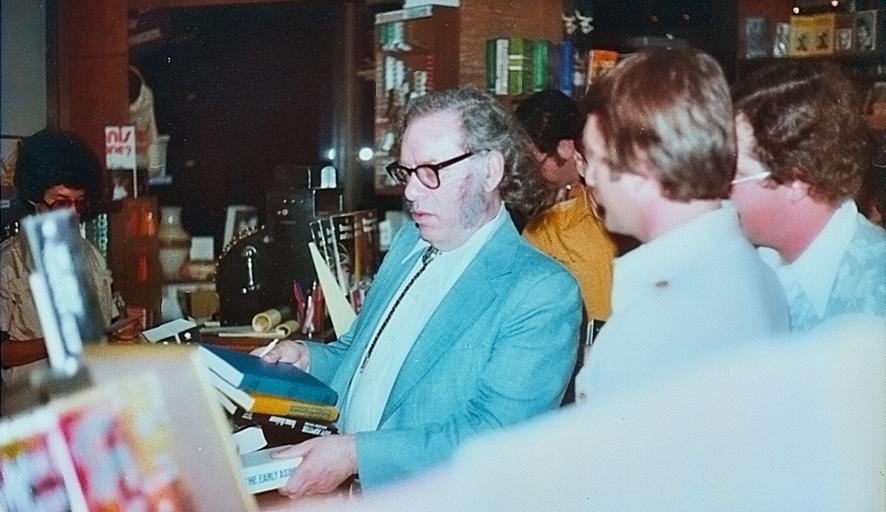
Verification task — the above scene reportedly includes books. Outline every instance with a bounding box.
[308,209,413,316]
[199,309,300,339]
[379,21,435,186]
[197,344,339,496]
[484,38,619,99]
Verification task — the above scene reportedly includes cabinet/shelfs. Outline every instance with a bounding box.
[719,0,886,102]
[346,0,563,213]
[48,0,219,334]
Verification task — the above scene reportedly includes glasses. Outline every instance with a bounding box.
[538,151,554,168]
[729,170,772,189]
[384,144,491,189]
[38,196,94,212]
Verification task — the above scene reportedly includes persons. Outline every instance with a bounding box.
[510,88,619,359]
[729,56,886,346]
[246,85,583,500]
[574,48,788,399]
[0,127,142,364]
